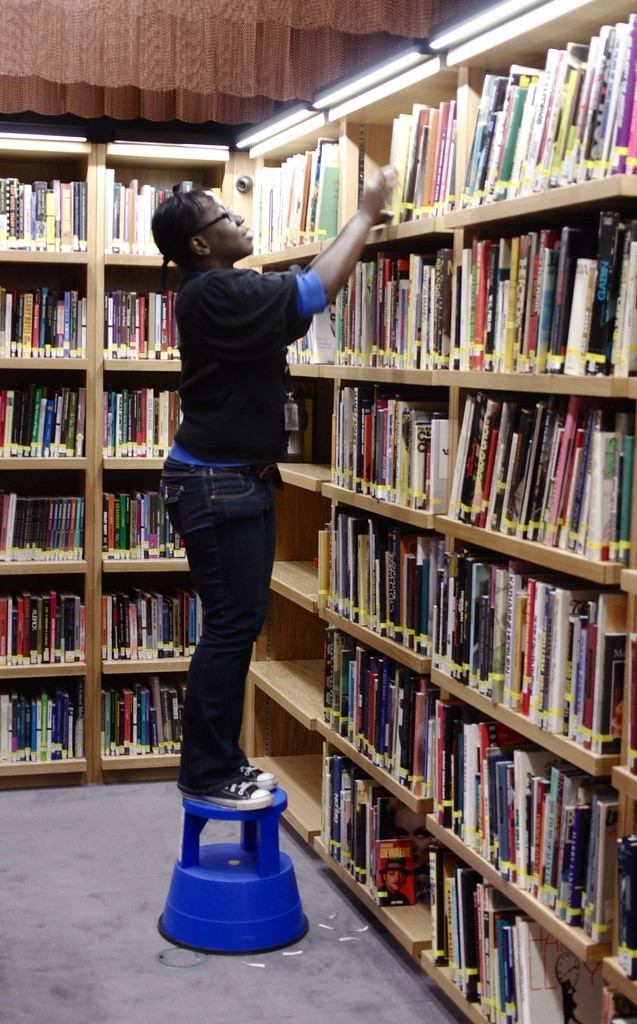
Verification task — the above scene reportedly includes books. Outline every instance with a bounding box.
[0,181,217,768]
[252,12,637,1024]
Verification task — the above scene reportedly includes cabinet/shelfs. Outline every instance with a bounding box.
[0,0,637,1024]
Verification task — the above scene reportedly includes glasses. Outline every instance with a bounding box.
[188,207,233,241]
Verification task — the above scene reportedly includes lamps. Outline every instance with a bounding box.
[311,38,431,112]
[234,101,318,150]
[425,0,548,51]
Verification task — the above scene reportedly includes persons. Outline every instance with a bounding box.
[137,175,391,811]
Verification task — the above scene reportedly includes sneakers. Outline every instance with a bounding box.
[234,764,278,792]
[182,779,274,811]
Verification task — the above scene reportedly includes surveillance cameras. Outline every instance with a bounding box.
[236,176,252,192]
